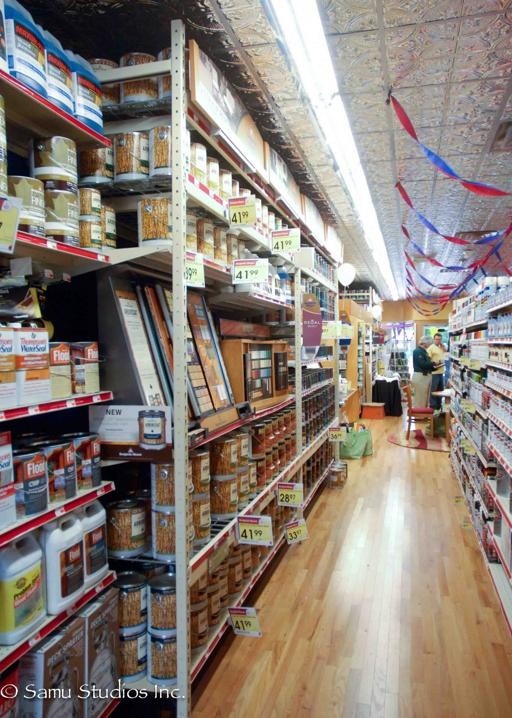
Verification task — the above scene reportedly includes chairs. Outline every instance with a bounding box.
[402,384,436,440]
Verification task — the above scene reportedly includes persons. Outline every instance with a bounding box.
[413,333,449,421]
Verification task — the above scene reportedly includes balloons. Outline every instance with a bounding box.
[372,305,381,318]
[337,263,356,286]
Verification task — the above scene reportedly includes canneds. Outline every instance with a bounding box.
[1,49,295,688]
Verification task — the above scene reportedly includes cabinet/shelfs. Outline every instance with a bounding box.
[446,284,512,623]
[0,12,387,714]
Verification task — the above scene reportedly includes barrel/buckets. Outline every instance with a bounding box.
[0,1,172,249]
[0,499,108,646]
